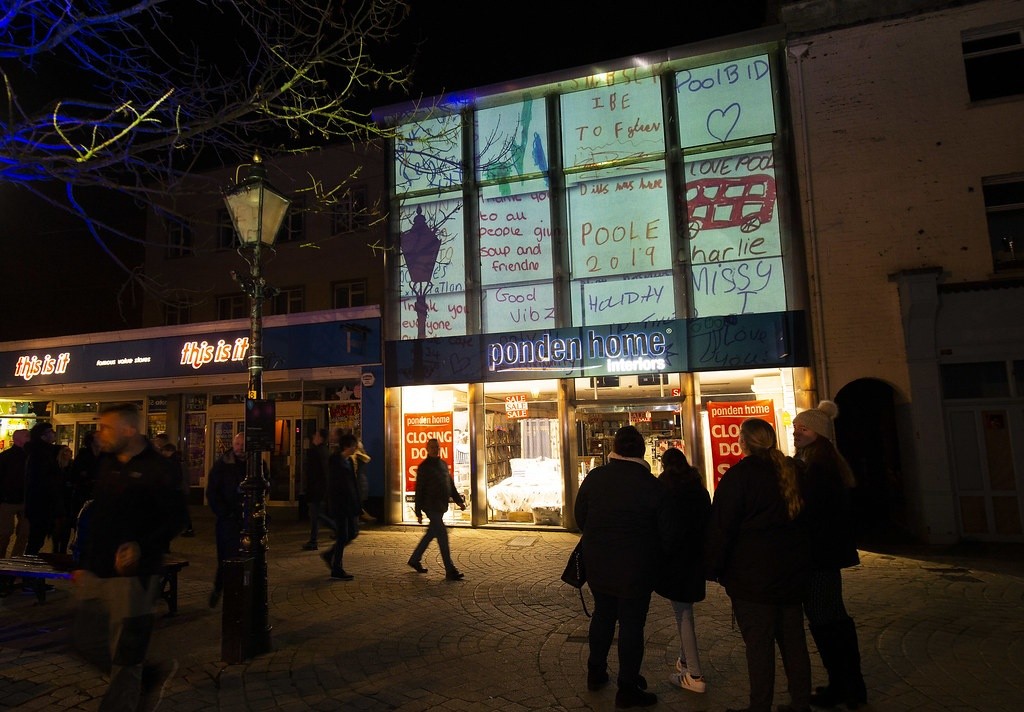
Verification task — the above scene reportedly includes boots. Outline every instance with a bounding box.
[809,616,867,710]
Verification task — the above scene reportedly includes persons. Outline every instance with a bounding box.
[0,402,196,662]
[793,399,867,711]
[703,419,830,712]
[319,434,365,581]
[206,432,270,609]
[654,447,712,693]
[574,425,684,712]
[72,403,191,712]
[302,432,337,550]
[407,439,466,580]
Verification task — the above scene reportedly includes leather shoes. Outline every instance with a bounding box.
[446,570,464,578]
[407,558,428,572]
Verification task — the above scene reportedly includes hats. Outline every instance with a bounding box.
[611,425,645,459]
[794,400,855,486]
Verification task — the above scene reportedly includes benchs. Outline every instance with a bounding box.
[0,551,189,619]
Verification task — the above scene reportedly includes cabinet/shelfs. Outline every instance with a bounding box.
[485,406,522,486]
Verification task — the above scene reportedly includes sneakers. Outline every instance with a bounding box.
[675,658,688,672]
[670,670,706,693]
[588,672,610,690]
[616,686,658,710]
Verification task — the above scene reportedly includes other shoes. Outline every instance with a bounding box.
[208,584,222,609]
[331,568,354,579]
[302,541,318,550]
[320,551,331,570]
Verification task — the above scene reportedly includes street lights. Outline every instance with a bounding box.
[224,149,293,652]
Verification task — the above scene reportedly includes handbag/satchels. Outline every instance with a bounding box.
[561,533,590,589]
